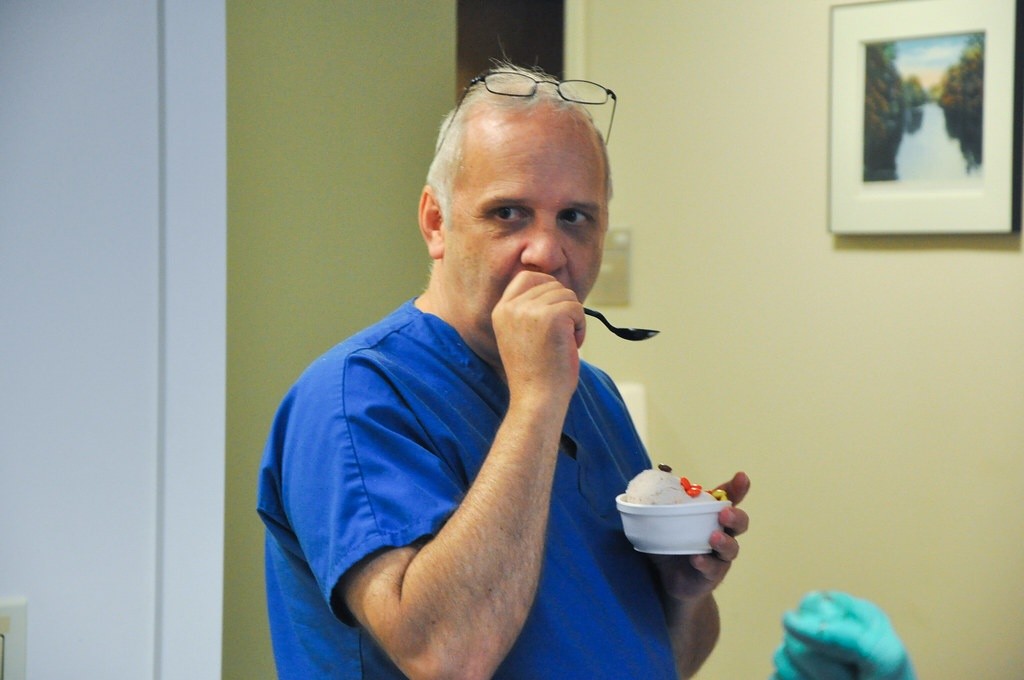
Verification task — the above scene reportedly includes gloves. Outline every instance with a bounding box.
[768,590,917,680]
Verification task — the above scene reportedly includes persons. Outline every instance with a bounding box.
[255,65,750,680]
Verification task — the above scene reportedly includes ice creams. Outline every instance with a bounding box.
[625,464,728,506]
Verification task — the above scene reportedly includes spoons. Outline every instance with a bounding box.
[582,307,660,341]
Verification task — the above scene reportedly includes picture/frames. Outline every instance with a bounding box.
[825,0,1024,237]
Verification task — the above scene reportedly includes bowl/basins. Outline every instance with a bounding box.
[615,493,731,555]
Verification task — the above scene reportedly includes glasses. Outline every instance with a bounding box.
[435,71,618,153]
